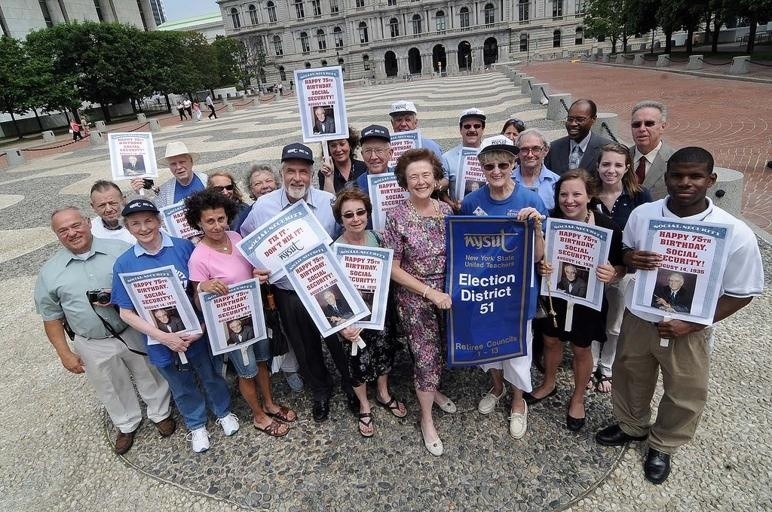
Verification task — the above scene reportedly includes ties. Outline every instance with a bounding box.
[239,335,242,341]
[634,156,646,184]
[167,325,172,332]
[569,283,573,292]
[569,145,580,167]
[670,292,675,300]
[322,125,325,132]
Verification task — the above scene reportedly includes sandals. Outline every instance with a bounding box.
[597,374,612,393]
[586,372,596,389]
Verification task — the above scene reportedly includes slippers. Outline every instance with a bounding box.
[265,404,297,422]
[358,412,374,437]
[376,397,407,418]
[254,419,290,437]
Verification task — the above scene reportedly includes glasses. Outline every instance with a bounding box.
[631,121,657,128]
[520,145,544,153]
[483,163,510,170]
[510,119,524,126]
[565,116,590,125]
[463,124,481,129]
[341,210,368,219]
[213,184,234,192]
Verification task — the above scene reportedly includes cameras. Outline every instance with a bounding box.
[85,288,111,304]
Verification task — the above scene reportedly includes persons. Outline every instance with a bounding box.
[554,261,586,297]
[143,97,162,107]
[386,100,450,195]
[123,154,145,175]
[246,79,294,101]
[155,308,184,335]
[534,165,625,432]
[592,145,766,486]
[382,148,459,458]
[460,133,549,439]
[322,290,352,325]
[109,197,243,453]
[87,177,168,245]
[311,106,337,133]
[325,189,408,437]
[176,92,221,122]
[317,124,367,193]
[33,204,177,457]
[341,125,400,197]
[500,120,525,144]
[509,129,560,211]
[68,116,93,141]
[439,107,495,213]
[543,97,616,178]
[626,100,670,203]
[239,142,360,425]
[238,163,306,392]
[471,180,479,193]
[183,189,298,438]
[225,319,254,346]
[205,170,251,234]
[586,139,653,397]
[154,139,211,209]
[650,268,692,314]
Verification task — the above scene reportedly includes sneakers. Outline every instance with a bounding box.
[215,412,240,436]
[283,372,304,392]
[185,424,212,453]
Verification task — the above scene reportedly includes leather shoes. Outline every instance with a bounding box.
[420,383,586,456]
[313,397,329,422]
[644,448,670,484]
[596,424,649,446]
[155,415,176,437]
[115,429,133,454]
[347,391,360,413]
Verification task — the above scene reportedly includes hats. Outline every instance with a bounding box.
[121,199,160,217]
[459,108,487,123]
[360,124,390,146]
[159,142,200,166]
[476,135,520,158]
[388,100,417,116]
[281,142,314,165]
